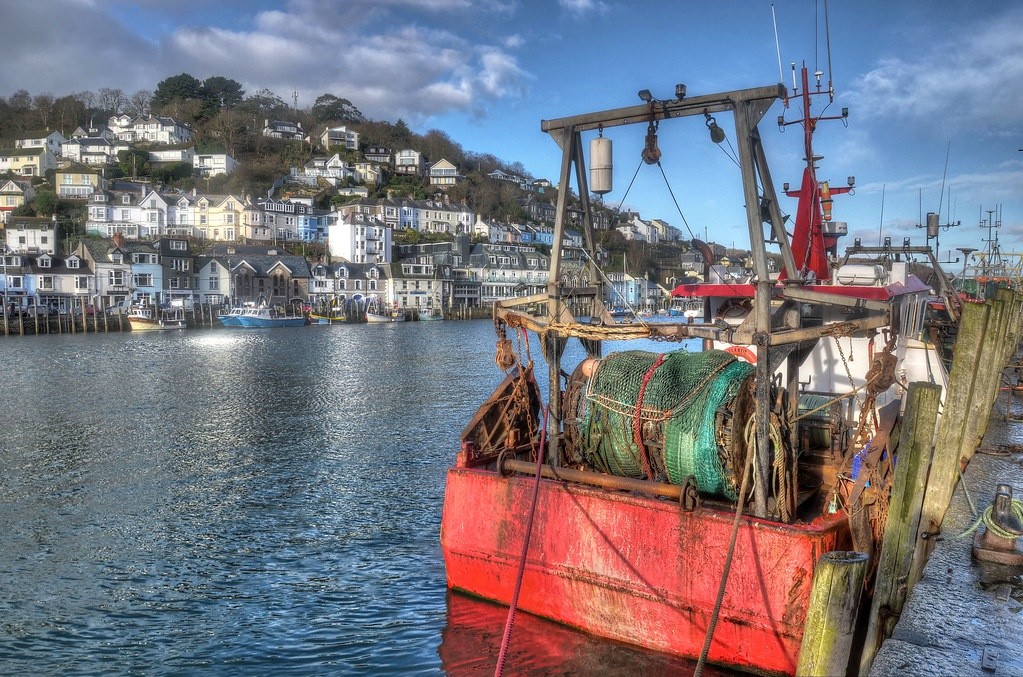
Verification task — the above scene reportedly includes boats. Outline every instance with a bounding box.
[366,307,405,325]
[657,305,704,319]
[126,306,187,332]
[439,2,1022,677]
[215,304,307,328]
[308,314,346,326]
[418,308,444,320]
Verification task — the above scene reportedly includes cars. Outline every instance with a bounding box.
[6,302,26,316]
[28,304,53,317]
[109,301,130,315]
[84,304,99,315]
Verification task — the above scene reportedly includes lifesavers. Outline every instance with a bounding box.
[725,345,758,366]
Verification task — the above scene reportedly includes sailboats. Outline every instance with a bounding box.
[636,270,654,318]
[611,252,632,317]
